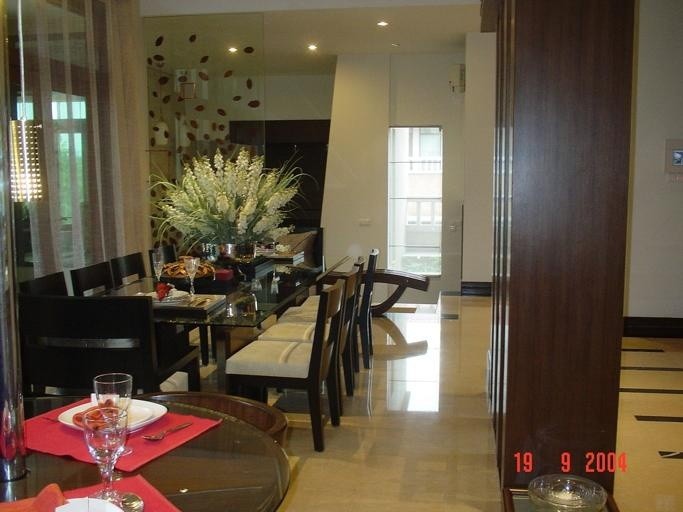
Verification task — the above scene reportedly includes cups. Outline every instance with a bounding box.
[152,249,163,282]
[205,244,220,281]
[84,406,126,504]
[94,373,131,457]
[183,258,199,300]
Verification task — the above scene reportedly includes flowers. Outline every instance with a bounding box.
[143,147,320,259]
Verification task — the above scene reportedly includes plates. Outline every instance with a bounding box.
[54,499,123,512]
[146,290,188,298]
[73,406,153,425]
[58,398,168,434]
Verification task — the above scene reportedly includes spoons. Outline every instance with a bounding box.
[119,492,143,512]
[142,422,193,441]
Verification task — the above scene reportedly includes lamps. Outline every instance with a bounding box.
[8,119,44,205]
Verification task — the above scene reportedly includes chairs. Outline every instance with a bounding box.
[19,293,201,397]
[223,277,346,451]
[69,261,113,296]
[110,252,146,288]
[148,245,176,277]
[278,255,366,385]
[256,264,359,415]
[18,271,68,295]
[300,248,380,369]
